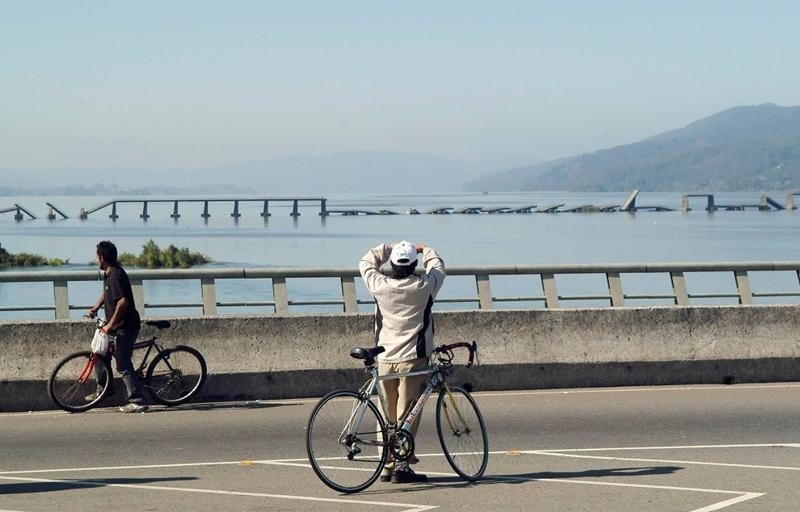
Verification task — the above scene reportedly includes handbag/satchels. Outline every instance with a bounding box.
[90,326,118,358]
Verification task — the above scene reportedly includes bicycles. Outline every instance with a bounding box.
[48,314,207,412]
[306,340,488,493]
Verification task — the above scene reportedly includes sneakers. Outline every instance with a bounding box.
[380,466,428,484]
[117,400,149,414]
[83,391,107,402]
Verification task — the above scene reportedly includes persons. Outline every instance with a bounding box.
[82,239,149,413]
[356,239,447,485]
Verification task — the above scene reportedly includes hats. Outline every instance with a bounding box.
[389,239,418,268]
